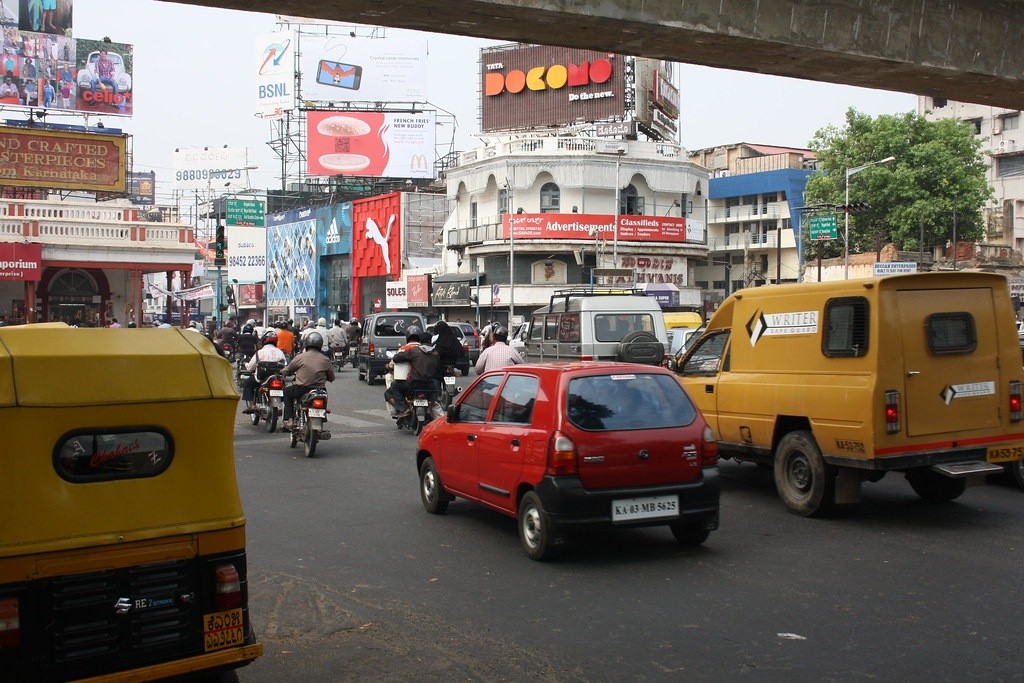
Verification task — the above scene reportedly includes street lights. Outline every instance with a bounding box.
[844,156,896,279]
[613,147,625,268]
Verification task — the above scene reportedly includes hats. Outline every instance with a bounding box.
[152,320,161,326]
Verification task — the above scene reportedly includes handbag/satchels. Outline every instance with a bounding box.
[257,362,285,380]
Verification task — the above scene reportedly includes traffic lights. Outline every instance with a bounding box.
[216,226,224,258]
[835,203,869,211]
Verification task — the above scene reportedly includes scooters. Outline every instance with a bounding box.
[210,329,362,389]
[384,363,464,437]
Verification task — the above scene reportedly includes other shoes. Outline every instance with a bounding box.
[399,408,409,419]
[392,410,401,419]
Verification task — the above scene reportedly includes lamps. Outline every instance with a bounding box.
[28,111,103,129]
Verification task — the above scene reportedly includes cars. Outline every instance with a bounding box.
[76,50,132,98]
[415,359,722,562]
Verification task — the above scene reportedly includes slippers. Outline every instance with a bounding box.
[282,423,293,433]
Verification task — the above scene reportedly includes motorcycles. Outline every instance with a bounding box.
[0,321,264,683]
[277,358,335,458]
[239,355,288,433]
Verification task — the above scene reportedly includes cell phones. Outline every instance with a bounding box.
[316,59,363,90]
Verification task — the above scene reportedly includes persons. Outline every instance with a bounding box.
[0,32,72,109]
[474,321,524,375]
[383,326,445,420]
[431,321,463,366]
[101,318,121,329]
[242,331,287,415]
[187,316,362,363]
[465,320,481,335]
[154,319,171,328]
[40,0,58,32]
[89,49,120,109]
[280,332,335,429]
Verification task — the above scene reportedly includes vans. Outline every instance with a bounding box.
[638,312,707,361]
[508,320,558,355]
[518,289,667,365]
[665,261,1024,517]
[353,312,425,386]
[426,322,480,366]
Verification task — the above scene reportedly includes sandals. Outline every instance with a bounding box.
[242,404,258,415]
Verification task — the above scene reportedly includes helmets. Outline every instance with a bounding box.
[246,319,256,327]
[350,318,358,326]
[305,332,323,352]
[494,326,509,336]
[262,331,278,347]
[281,322,287,329]
[303,321,308,326]
[273,321,283,329]
[243,324,254,336]
[406,326,423,343]
[188,320,196,328]
[487,320,492,324]
[492,323,501,332]
[286,319,294,325]
[318,318,326,327]
[307,321,314,327]
